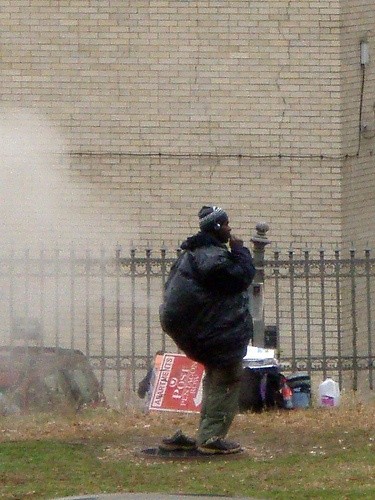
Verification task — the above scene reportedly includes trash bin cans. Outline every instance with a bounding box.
[238,346,282,409]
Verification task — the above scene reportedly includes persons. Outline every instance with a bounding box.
[186,205,255,454]
[137,350,167,398]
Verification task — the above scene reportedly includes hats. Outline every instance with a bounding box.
[198,206,228,232]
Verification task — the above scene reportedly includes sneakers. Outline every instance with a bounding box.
[198,438,241,456]
[160,430,198,450]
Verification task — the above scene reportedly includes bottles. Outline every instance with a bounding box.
[319,376,340,409]
[281,379,294,410]
